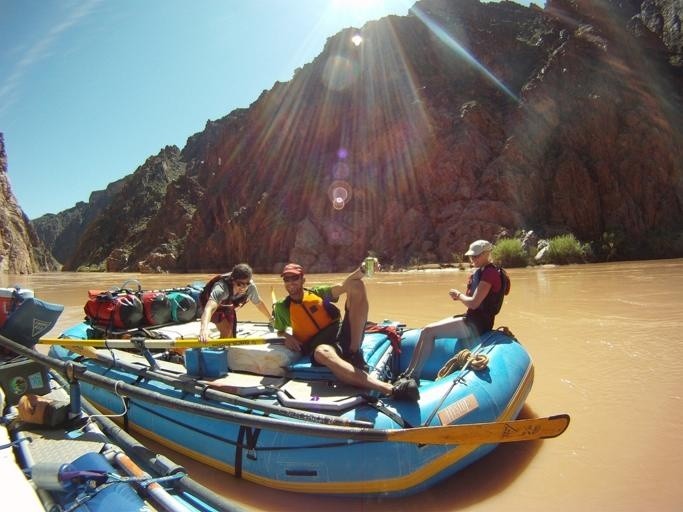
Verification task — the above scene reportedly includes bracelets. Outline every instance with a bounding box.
[457,292,464,300]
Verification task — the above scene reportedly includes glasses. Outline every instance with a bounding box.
[237,282,251,287]
[283,276,299,281]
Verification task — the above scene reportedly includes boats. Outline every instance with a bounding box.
[9,346,259,512]
[47,314,534,498]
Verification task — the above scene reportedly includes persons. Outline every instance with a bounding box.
[272,257,421,403]
[397,239,512,387]
[198,263,272,345]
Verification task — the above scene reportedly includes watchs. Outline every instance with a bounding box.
[360,266,365,273]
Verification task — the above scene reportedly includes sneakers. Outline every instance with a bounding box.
[397,368,409,378]
[348,348,369,372]
[406,369,420,386]
[392,380,419,401]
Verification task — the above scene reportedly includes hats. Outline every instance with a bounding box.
[465,240,492,255]
[281,263,304,276]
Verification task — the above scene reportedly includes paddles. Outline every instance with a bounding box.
[0,336,569,445]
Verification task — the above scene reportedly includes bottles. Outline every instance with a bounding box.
[365,257,374,277]
